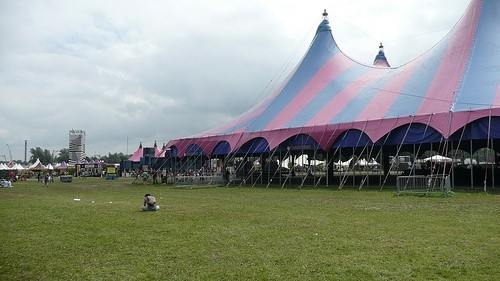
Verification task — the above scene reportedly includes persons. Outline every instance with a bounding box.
[0,167,227,188]
[140,194,158,211]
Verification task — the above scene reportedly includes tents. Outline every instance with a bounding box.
[0,0,500,171]
